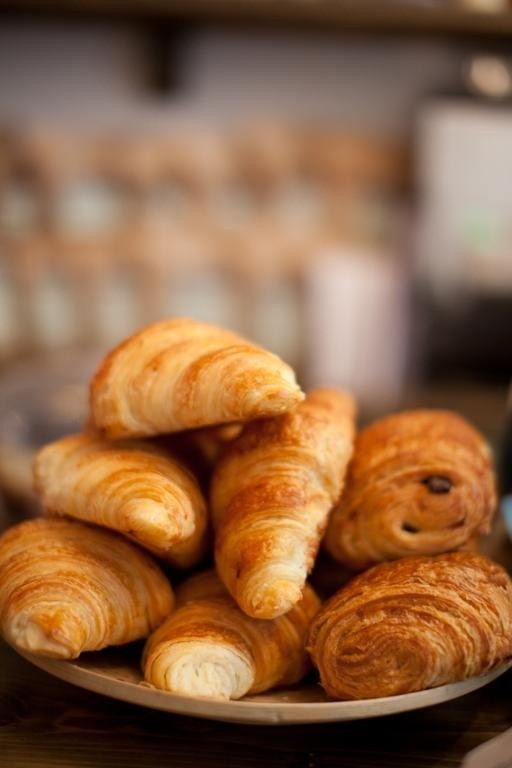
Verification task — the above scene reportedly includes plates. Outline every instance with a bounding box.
[3,636,512,727]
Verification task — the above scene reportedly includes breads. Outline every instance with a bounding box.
[32,432,209,569]
[303,554,511,700]
[1,516,178,662]
[205,386,358,621]
[86,320,306,444]
[141,572,321,699]
[319,409,497,569]
